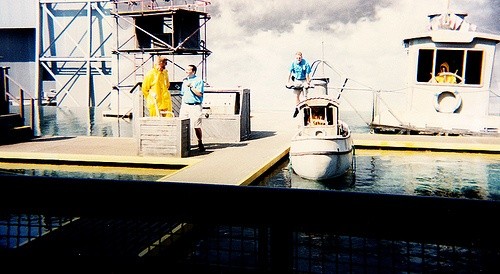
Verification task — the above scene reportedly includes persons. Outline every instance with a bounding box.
[436,63,457,86]
[179,64,205,153]
[287,51,312,118]
[141,57,172,118]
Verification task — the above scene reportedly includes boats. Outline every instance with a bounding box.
[287,27,354,184]
[351,0,494,153]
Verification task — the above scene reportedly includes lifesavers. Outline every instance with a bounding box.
[433,86,462,114]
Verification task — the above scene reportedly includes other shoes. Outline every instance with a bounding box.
[197,143,206,152]
[294,107,299,117]
